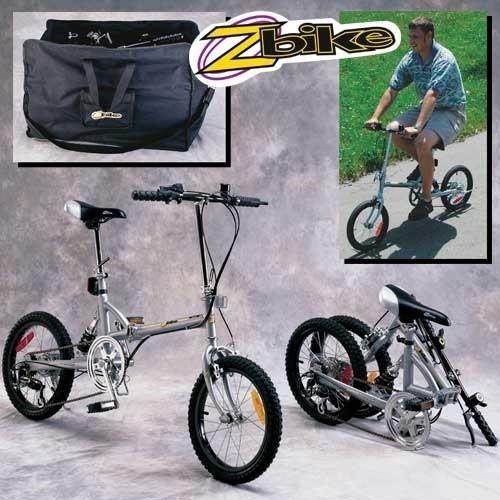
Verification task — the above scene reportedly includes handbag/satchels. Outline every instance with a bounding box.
[22,19,213,153]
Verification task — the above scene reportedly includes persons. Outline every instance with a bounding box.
[365,15,467,224]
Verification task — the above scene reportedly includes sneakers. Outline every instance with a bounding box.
[409,197,433,219]
[407,164,420,180]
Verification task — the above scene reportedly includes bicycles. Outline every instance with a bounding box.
[343,118,475,258]
[2,177,286,488]
[285,275,499,455]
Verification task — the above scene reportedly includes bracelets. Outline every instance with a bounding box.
[413,125,422,131]
